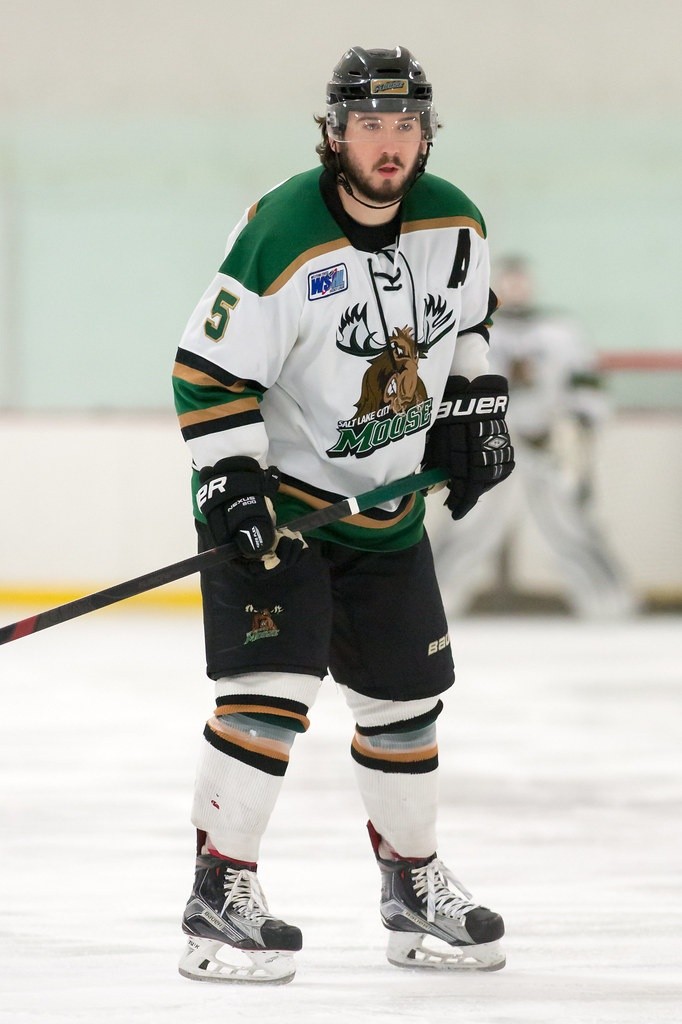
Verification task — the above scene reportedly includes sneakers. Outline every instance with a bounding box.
[178,829,302,986]
[366,820,506,972]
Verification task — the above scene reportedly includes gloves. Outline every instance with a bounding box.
[421,375,516,521]
[196,455,309,581]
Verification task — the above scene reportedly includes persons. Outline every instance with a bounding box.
[172,46,515,986]
[434,253,638,625]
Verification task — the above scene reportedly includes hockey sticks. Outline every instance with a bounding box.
[0,463,453,647]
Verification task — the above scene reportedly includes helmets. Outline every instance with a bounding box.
[326,46,437,134]
